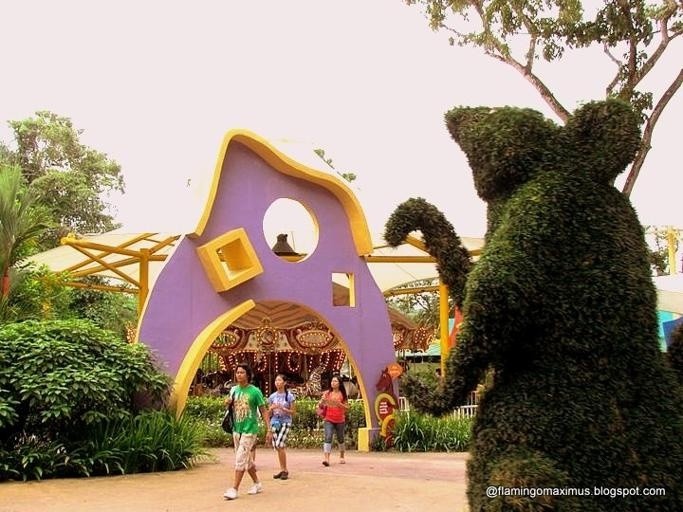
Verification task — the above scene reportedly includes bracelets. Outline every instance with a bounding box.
[266,429,271,432]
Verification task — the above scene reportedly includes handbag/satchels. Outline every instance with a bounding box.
[221,392,235,434]
[316,404,327,417]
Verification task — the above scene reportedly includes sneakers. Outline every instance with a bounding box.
[248,483,262,494]
[339,458,345,463]
[323,461,329,466]
[224,488,238,499]
[273,471,288,480]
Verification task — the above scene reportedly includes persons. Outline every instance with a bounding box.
[317,375,349,466]
[287,366,327,400]
[265,374,295,480]
[222,363,273,499]
